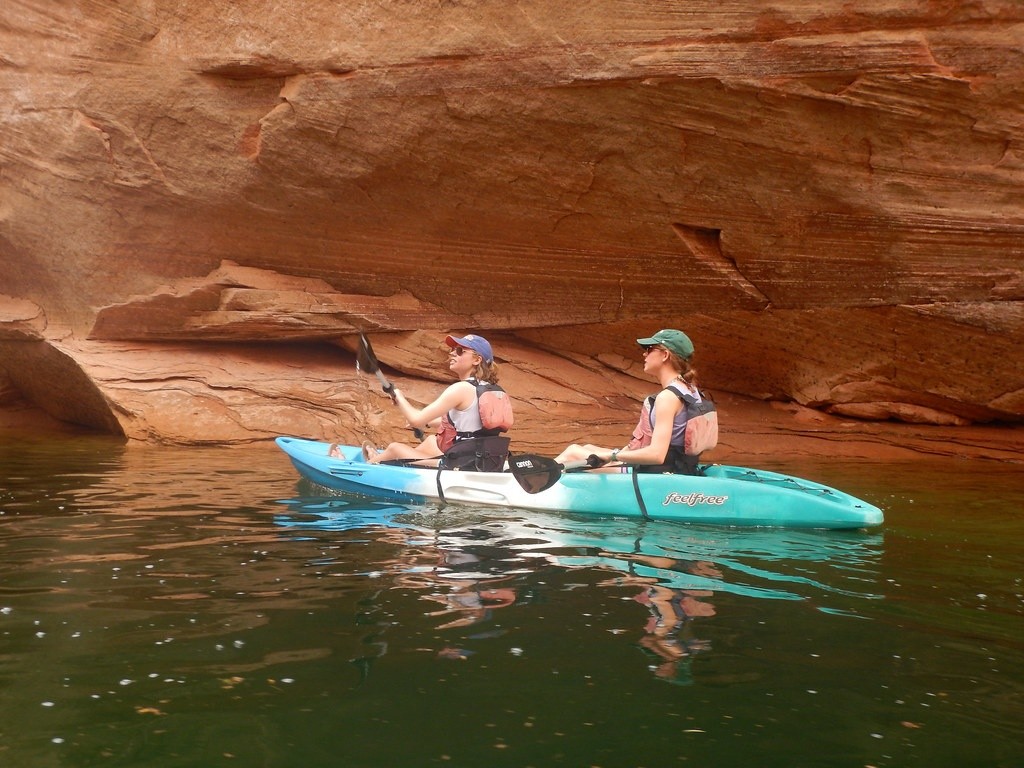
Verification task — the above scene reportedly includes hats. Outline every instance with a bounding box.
[635,329,695,362]
[445,334,495,366]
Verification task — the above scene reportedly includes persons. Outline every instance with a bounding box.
[554,328,702,479]
[328,332,498,474]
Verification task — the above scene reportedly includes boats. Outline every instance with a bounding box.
[275,438,884,529]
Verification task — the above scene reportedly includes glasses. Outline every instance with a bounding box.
[646,345,668,354]
[450,346,474,356]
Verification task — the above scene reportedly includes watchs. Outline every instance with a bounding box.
[612,447,620,461]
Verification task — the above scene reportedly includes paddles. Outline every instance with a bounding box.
[355,330,428,445]
[507,453,605,495]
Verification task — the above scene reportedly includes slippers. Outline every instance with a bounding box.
[328,442,340,457]
[362,440,377,462]
